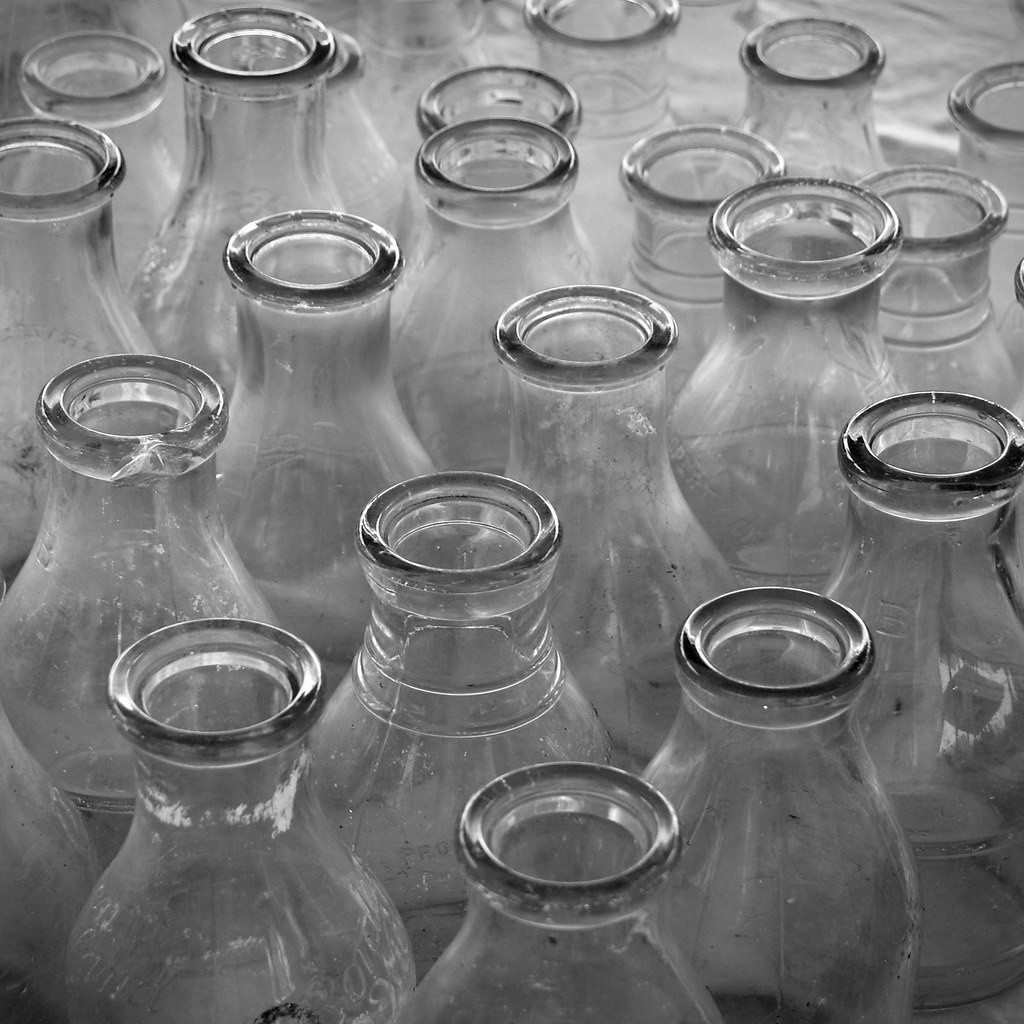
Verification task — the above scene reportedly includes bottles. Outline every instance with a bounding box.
[6,5,1023,1022]
